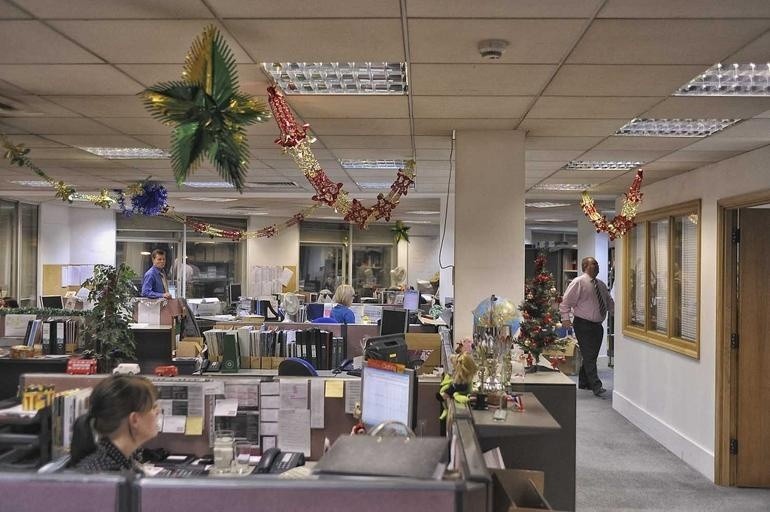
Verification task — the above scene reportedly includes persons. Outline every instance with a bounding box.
[560,257,614,394]
[327,274,335,286]
[65,374,160,477]
[142,249,172,299]
[364,269,376,286]
[330,285,355,323]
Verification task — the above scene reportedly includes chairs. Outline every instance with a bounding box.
[277,357,318,377]
[312,317,340,323]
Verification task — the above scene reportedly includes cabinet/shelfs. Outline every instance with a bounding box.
[454,339,577,512]
[560,247,577,296]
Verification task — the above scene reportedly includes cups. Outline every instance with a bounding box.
[212,428,250,473]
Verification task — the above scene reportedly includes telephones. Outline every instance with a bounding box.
[339,359,361,372]
[201,359,222,372]
[252,449,305,474]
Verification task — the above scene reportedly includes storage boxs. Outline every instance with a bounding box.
[488,468,552,512]
[541,336,582,376]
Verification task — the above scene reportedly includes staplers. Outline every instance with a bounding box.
[144,449,163,462]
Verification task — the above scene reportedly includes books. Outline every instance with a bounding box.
[203,326,345,371]
[24,319,76,355]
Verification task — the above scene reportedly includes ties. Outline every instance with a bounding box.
[160,272,169,293]
[593,280,607,317]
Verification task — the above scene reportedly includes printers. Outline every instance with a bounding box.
[186,298,220,316]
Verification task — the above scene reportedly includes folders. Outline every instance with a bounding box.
[296,327,332,370]
[42,319,67,355]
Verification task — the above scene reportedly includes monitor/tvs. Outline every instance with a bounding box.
[40,295,64,309]
[380,307,408,336]
[230,283,241,304]
[361,360,417,430]
[193,282,215,297]
[404,290,419,314]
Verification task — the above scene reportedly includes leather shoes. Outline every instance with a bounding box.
[593,387,606,395]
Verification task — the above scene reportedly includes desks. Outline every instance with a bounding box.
[0,396,52,474]
[196,319,216,328]
[127,323,172,376]
[0,353,69,399]
